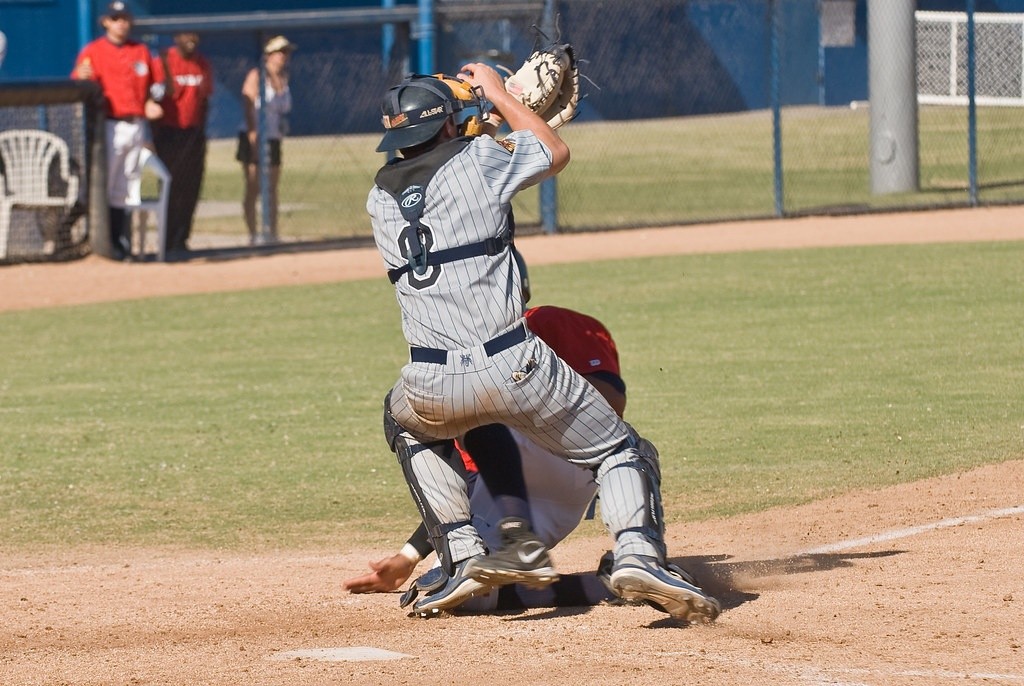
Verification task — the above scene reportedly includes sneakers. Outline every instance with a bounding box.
[413,553,495,612]
[596,552,697,614]
[467,517,559,590]
[611,554,721,625]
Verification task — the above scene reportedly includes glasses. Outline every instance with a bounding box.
[106,14,130,20]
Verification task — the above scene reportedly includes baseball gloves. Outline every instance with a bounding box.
[503,40,581,129]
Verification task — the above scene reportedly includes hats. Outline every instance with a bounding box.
[265,35,298,53]
[100,2,132,17]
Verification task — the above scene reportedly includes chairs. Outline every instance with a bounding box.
[0,129,173,263]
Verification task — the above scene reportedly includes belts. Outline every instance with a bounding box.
[411,322,527,365]
[107,114,146,123]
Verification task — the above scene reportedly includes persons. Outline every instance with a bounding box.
[345,64,722,629]
[69,0,214,263]
[236,36,297,246]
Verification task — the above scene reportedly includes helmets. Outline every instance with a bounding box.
[430,73,483,137]
[372,73,463,153]
